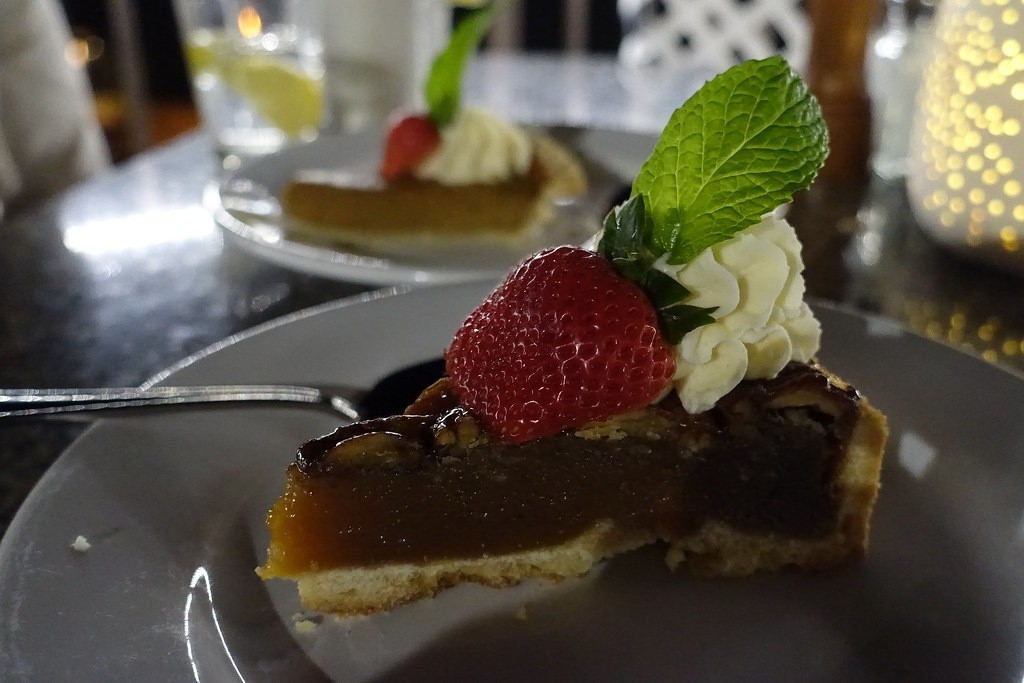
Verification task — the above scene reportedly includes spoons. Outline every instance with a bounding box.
[1,355,446,425]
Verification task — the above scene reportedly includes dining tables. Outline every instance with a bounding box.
[0,116,1024,683]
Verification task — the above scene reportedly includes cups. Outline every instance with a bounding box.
[173,0,327,154]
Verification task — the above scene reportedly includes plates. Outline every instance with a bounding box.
[0,270,1024,683]
[207,124,661,288]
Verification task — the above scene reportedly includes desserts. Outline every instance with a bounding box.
[256,55,894,619]
[278,3,583,248]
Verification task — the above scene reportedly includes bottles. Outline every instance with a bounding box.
[785,0,878,281]
